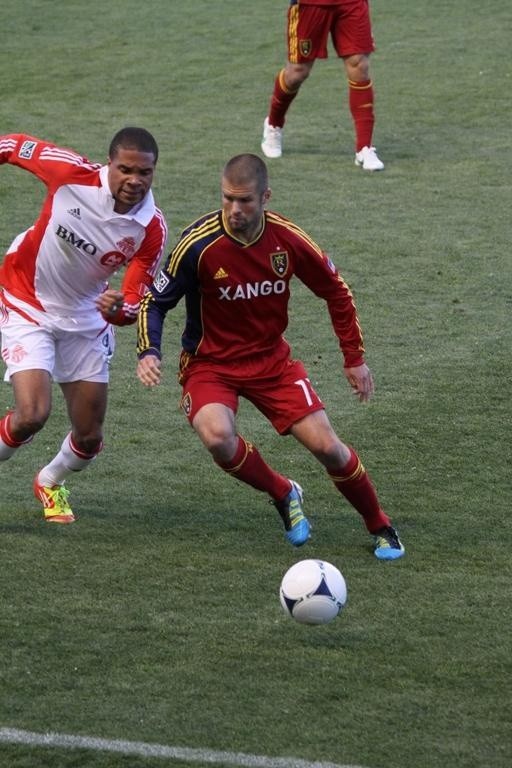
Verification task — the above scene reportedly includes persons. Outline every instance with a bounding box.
[0,128,168,526]
[259,0,390,172]
[137,153,407,563]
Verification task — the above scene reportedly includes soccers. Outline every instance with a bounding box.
[278,559,347,627]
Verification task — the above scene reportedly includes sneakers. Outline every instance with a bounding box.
[374,525,404,561]
[33,475,74,525]
[354,146,383,172]
[275,477,311,547]
[261,115,282,159]
